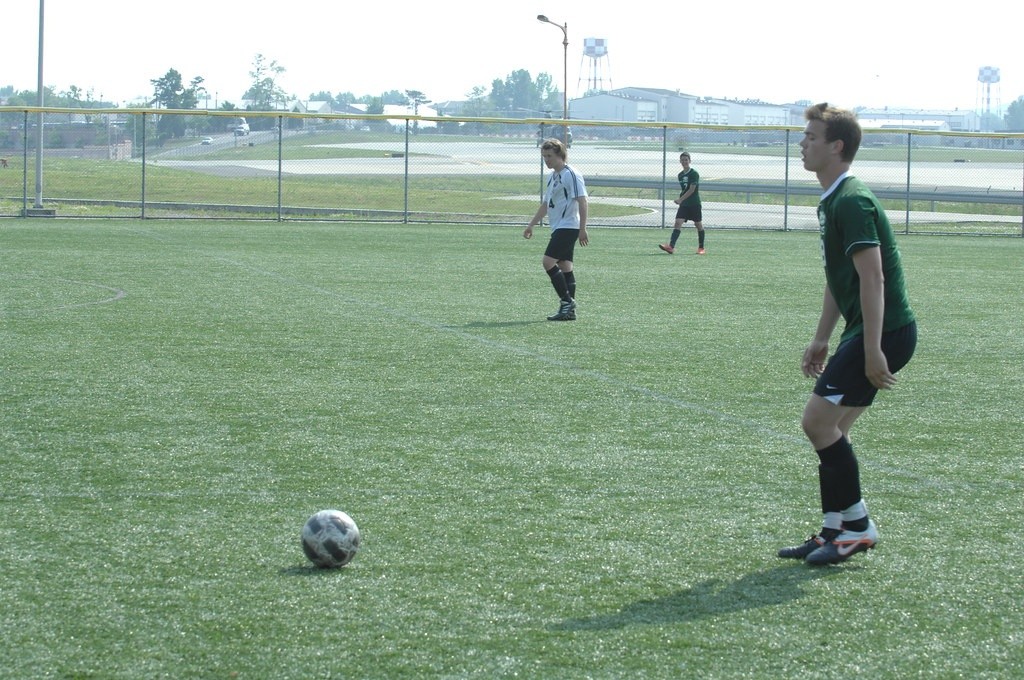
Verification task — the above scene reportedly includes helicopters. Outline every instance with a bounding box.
[489,107,578,149]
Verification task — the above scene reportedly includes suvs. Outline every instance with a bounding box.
[233,125,249,136]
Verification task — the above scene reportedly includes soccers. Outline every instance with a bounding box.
[300,509,360,569]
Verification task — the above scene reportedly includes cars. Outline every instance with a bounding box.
[202,137,213,144]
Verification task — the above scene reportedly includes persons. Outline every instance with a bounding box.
[777,104,918,566]
[659,153,706,255]
[524,138,589,320]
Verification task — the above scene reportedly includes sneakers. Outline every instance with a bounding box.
[807,519,875,566]
[659,244,674,254]
[696,248,705,254]
[778,534,827,559]
[547,298,576,321]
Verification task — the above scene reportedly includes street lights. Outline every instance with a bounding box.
[538,15,567,149]
